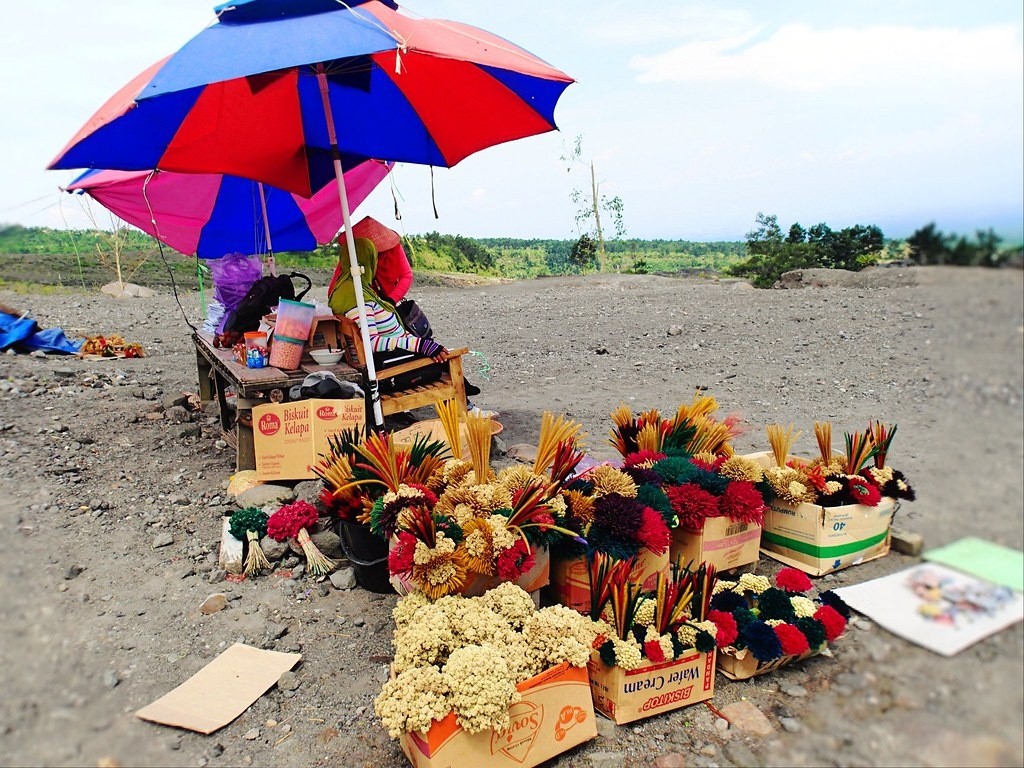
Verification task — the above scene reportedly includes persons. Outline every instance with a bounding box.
[327,239,499,419]
[326,215,413,305]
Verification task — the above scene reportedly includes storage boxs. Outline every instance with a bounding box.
[666,506,762,575]
[262,314,341,363]
[742,448,896,578]
[387,654,599,768]
[386,529,552,614]
[583,637,719,726]
[546,536,669,616]
[251,398,367,481]
[715,638,830,682]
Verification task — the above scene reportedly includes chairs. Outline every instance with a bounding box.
[327,313,469,431]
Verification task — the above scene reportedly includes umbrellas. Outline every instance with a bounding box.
[43,0,576,434]
[59,157,396,278]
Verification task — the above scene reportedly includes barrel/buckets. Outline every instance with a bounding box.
[396,300,430,339]
[268,335,316,371]
[337,520,400,594]
[274,297,316,340]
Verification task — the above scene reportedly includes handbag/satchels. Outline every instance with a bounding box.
[396,301,433,340]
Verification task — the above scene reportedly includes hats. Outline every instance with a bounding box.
[300,370,355,401]
[339,215,400,253]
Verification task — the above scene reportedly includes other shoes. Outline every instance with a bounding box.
[463,378,480,397]
[467,403,498,420]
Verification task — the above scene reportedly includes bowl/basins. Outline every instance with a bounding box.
[308,348,345,366]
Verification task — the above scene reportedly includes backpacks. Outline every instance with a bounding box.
[224,272,311,334]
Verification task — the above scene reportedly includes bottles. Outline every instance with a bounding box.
[243,331,268,369]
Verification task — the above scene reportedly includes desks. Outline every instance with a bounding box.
[191,330,364,473]
